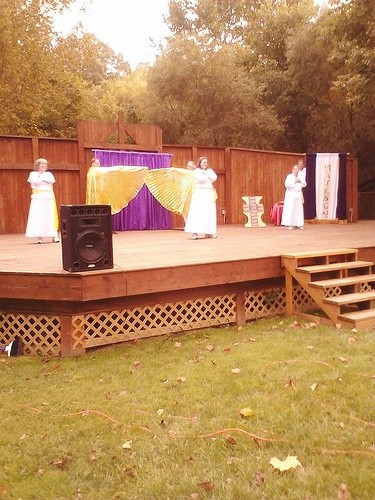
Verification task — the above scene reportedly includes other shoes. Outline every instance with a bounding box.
[285,225,294,231]
[206,234,218,240]
[36,237,44,244]
[192,233,199,240]
[297,226,304,230]
[53,236,60,242]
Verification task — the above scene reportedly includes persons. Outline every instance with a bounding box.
[25,154,61,246]
[295,159,307,191]
[89,158,100,167]
[183,155,218,240]
[185,159,197,169]
[279,163,305,230]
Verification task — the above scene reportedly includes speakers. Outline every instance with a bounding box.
[60,204,114,273]
